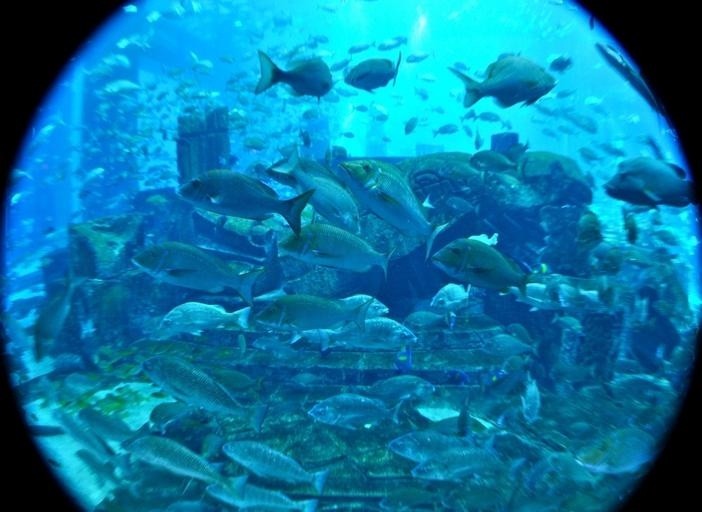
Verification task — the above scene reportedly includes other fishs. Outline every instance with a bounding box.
[1,0,701,512]
